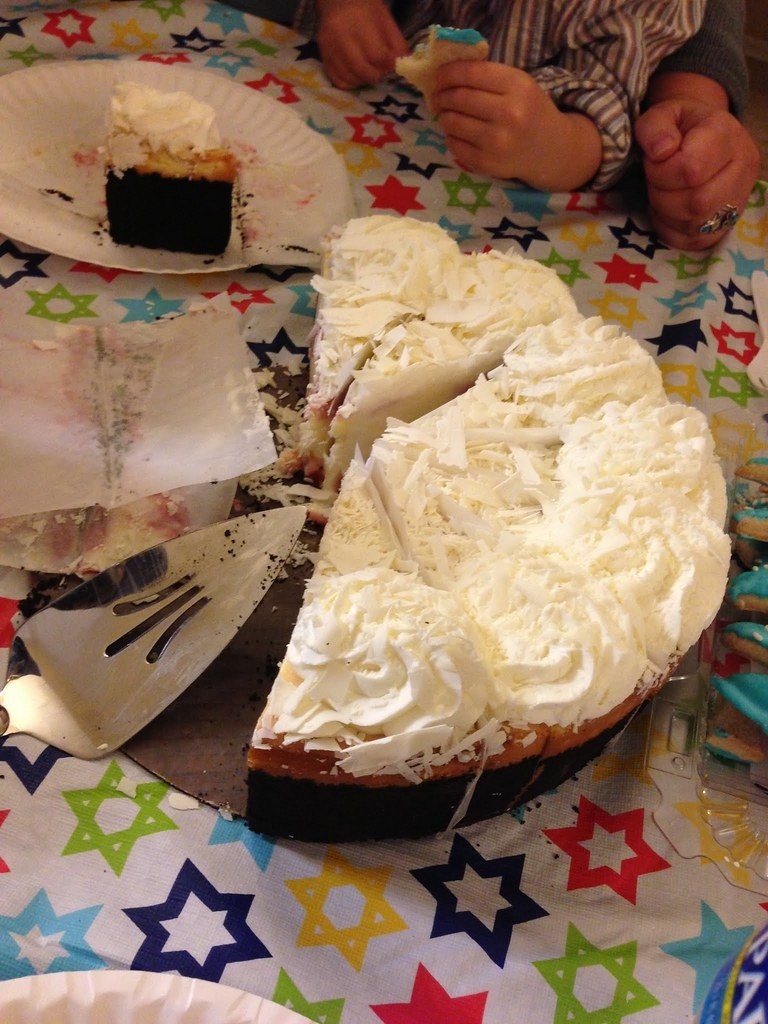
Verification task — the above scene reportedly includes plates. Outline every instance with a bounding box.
[0,970,316,1024]
[0,58,352,276]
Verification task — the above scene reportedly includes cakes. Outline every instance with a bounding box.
[104,81,238,255]
[244,213,735,846]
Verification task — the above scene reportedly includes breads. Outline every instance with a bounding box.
[702,456,768,766]
[392,24,490,117]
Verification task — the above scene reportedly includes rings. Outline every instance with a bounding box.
[701,201,739,233]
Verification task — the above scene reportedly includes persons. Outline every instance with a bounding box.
[637,0,762,252]
[319,0,705,197]
